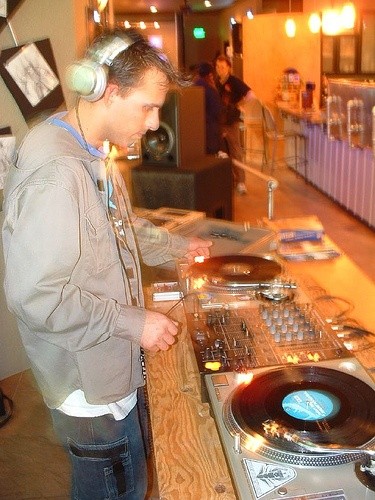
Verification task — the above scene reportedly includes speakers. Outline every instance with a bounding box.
[129,85,235,223]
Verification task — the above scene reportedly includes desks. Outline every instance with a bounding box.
[272,100,374,232]
[145,216,375,500]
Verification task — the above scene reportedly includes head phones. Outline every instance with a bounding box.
[68,34,141,102]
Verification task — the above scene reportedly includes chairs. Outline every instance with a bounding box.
[242,105,309,184]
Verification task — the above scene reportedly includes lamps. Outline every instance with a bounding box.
[285,0,320,37]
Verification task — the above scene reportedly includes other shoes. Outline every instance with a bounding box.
[236,183,246,194]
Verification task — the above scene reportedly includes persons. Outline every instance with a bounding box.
[195,54,254,196]
[0,27,208,500]
